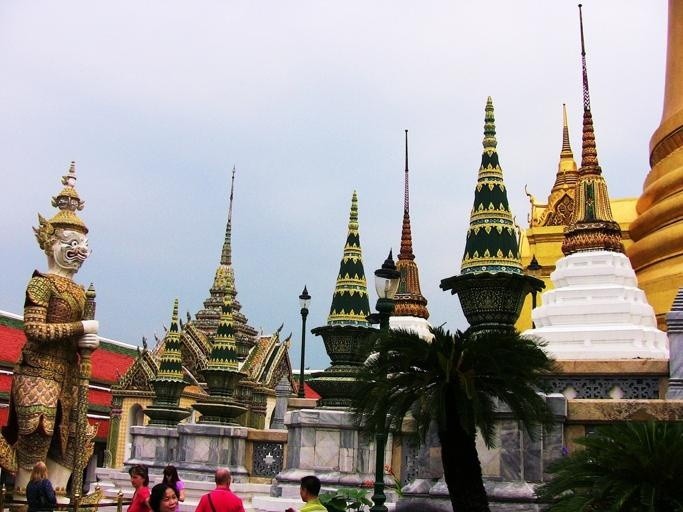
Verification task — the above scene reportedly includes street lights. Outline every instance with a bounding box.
[298,283,311,398]
[369,247,401,511]
[526,255,542,329]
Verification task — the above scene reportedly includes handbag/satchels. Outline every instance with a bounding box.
[38,496,50,505]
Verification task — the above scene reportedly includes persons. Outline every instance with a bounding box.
[286,476,328,512]
[127,465,185,512]
[26,461,56,512]
[195,468,246,512]
